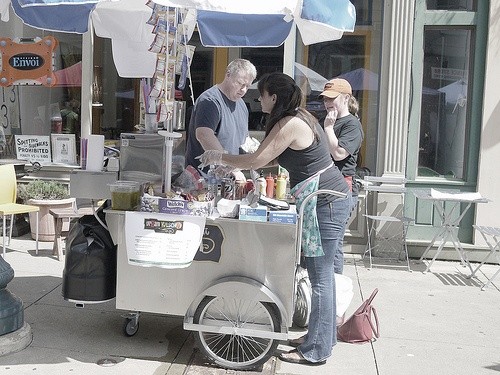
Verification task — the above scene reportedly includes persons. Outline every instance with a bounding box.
[194,72,353,364]
[315,77,365,274]
[184,58,258,184]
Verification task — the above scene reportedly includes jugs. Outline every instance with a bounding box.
[144,112,158,134]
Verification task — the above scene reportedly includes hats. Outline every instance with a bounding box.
[319,78,352,98]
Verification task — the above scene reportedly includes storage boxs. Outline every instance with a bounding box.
[269,204,297,224]
[239,204,267,223]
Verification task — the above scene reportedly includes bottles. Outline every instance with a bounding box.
[256,169,288,200]
[245,179,254,193]
[207,165,218,196]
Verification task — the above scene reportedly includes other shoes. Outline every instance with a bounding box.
[279,348,326,365]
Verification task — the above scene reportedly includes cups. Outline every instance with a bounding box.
[50,116,62,134]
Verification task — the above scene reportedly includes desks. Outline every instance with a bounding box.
[410,186,494,276]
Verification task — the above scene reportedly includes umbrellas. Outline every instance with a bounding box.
[10,0,356,194]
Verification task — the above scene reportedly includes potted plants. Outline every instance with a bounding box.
[16,180,77,242]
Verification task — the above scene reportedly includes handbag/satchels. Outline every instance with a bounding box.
[337,288,380,343]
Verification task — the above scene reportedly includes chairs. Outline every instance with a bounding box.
[359,174,417,273]
[466,224,500,290]
[0,164,42,256]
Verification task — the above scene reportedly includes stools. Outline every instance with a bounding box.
[48,207,99,262]
